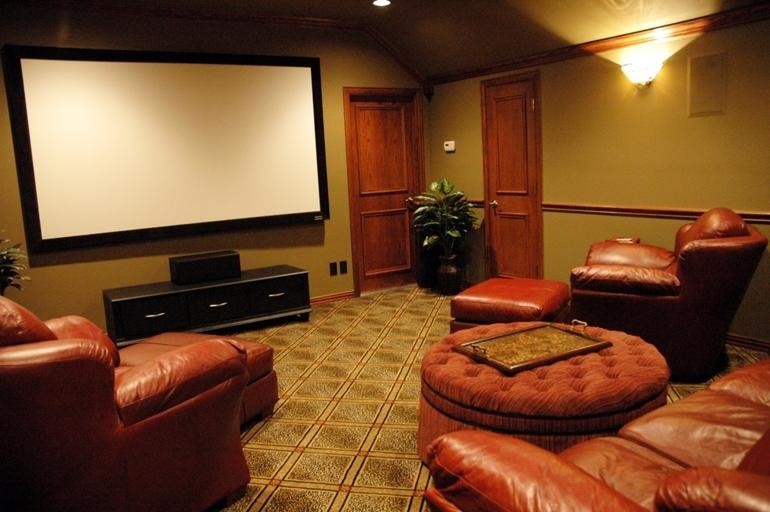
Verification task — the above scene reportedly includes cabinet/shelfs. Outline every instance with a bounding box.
[102,263,312,347]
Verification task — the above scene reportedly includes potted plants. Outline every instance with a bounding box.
[406,177,478,296]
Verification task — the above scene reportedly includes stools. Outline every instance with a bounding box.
[450,276,572,332]
[232,333,279,428]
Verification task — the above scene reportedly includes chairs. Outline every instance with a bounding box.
[0,295,251,511]
[572,207,769,385]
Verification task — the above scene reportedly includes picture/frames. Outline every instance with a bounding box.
[454,321,612,375]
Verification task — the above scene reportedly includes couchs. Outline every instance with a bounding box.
[425,358,769,512]
